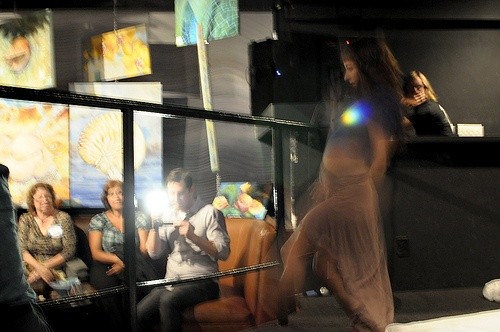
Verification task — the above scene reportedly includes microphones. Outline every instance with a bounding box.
[294,287,330,297]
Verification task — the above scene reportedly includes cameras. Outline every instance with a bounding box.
[156,219,177,229]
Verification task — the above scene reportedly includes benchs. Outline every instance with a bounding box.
[181,219,276,331]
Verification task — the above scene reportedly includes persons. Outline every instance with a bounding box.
[18,182,89,291]
[399,70,453,138]
[263,35,396,332]
[87,181,161,332]
[136,169,231,332]
[0,163,54,332]
